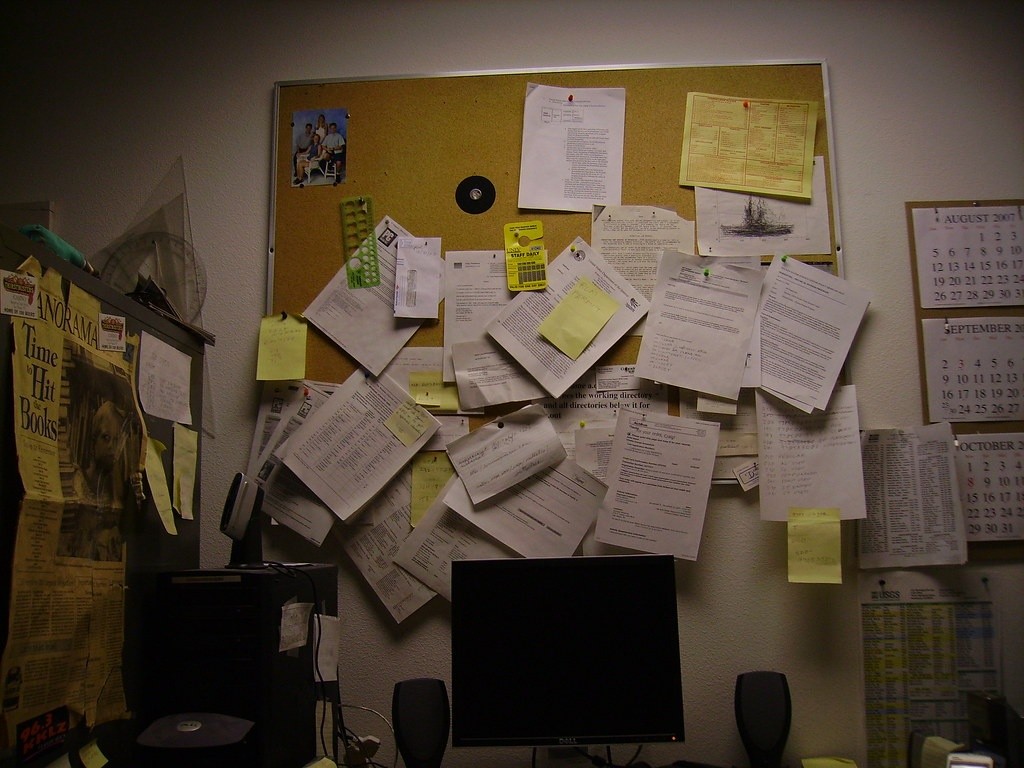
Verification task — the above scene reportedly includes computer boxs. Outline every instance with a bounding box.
[119,563,338,768]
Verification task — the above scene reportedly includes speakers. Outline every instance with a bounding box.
[392,678,451,768]
[220,472,268,571]
[735,671,792,768]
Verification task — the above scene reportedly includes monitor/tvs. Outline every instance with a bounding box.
[450,554,685,768]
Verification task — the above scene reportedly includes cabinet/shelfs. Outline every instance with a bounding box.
[1,225,216,767]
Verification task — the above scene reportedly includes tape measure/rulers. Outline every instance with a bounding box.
[79,156,217,442]
[339,194,380,290]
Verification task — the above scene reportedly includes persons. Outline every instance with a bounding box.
[53,517,82,558]
[290,113,346,185]
[75,401,131,513]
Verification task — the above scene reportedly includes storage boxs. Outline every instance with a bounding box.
[1,201,66,241]
